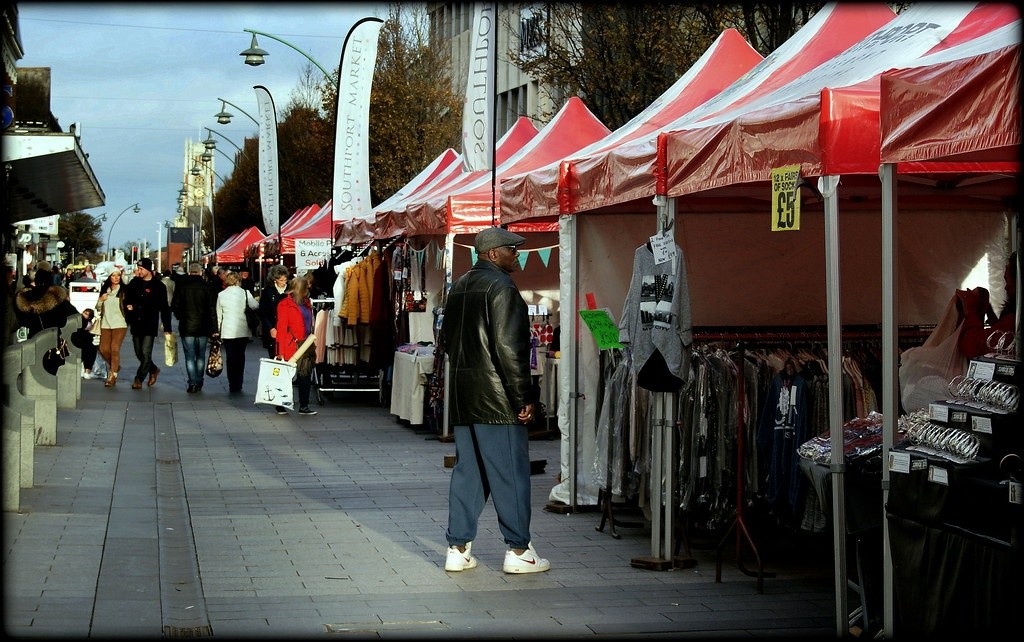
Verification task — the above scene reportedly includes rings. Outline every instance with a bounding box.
[528,414,531,418]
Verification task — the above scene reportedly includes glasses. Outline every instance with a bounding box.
[503,245,517,255]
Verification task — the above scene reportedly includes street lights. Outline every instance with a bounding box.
[107,203,141,262]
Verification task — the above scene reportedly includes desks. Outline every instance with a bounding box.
[390,351,452,437]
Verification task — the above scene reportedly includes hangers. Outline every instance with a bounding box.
[647,220,674,237]
[318,302,336,314]
[354,241,376,265]
[608,323,940,365]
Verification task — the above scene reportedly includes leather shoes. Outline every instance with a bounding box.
[132,378,142,389]
[148,367,160,386]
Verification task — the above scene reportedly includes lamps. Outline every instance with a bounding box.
[239,29,338,91]
[201,148,241,171]
[213,98,260,128]
[189,159,226,185]
[201,127,250,163]
[92,212,107,222]
[107,203,141,251]
[176,184,209,227]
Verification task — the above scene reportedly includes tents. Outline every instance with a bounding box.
[656,2,1024,642]
[202,27,766,642]
[882,2,1024,642]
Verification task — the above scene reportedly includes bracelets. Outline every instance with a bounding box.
[908,422,980,460]
[947,375,1019,412]
[998,331,1016,350]
[999,454,1023,476]
[987,330,1006,350]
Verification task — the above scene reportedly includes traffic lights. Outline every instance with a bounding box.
[113,248,116,257]
[134,247,137,261]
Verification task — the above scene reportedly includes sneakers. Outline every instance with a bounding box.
[105,371,118,386]
[503,541,550,573]
[445,542,477,571]
[276,408,288,415]
[299,407,317,415]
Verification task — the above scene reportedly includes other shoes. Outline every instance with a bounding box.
[197,384,202,391]
[84,372,98,379]
[187,384,198,393]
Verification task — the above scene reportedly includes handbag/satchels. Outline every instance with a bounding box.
[206,336,223,378]
[48,338,69,363]
[253,356,297,411]
[164,332,178,367]
[898,294,969,415]
[89,318,102,335]
[296,339,317,377]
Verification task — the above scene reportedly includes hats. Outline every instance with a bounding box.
[137,258,152,272]
[108,267,121,278]
[474,228,527,253]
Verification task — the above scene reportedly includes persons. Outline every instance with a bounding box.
[13,257,318,416]
[958,250,1016,357]
[436,226,550,574]
